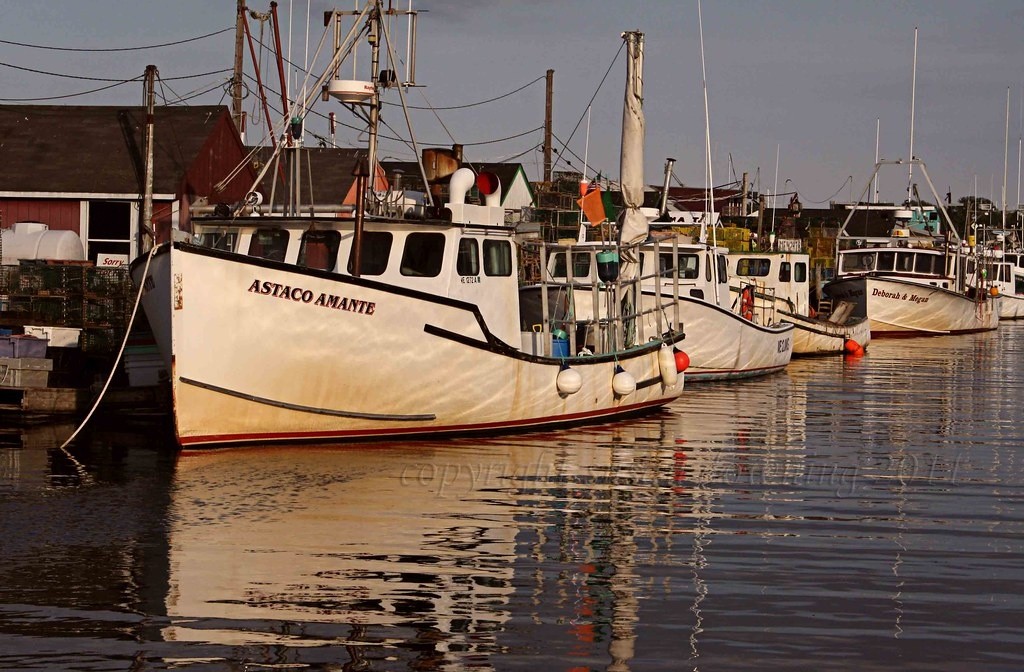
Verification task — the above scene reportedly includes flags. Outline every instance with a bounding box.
[577,188,615,227]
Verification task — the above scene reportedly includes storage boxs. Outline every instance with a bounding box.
[24,325,83,347]
[0,337,50,359]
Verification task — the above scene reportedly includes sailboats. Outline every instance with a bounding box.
[126,0,691,444]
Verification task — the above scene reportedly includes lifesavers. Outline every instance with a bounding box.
[740,287,754,322]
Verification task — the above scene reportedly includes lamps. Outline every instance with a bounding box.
[379,69,395,82]
[856,240,862,249]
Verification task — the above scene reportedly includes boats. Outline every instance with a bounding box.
[521,153,872,381]
[819,29,1024,335]
